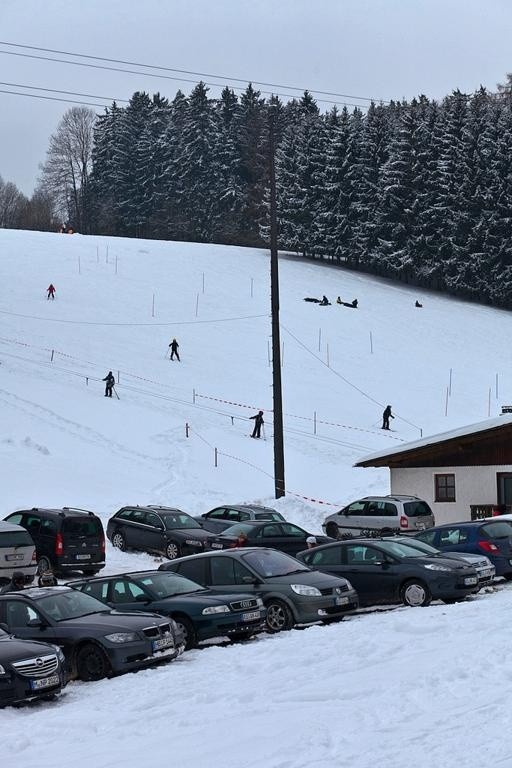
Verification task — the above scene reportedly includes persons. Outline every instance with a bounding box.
[47,283,55,300]
[249,411,264,438]
[415,300,422,307]
[381,404,395,430]
[336,297,343,304]
[102,371,115,398]
[0,571,30,626]
[169,338,180,361]
[38,570,58,586]
[321,296,328,305]
[306,536,320,549]
[352,298,358,308]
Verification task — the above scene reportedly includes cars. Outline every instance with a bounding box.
[296,541,478,607]
[472,512,512,528]
[204,519,335,559]
[414,520,512,582]
[0,624,69,709]
[351,534,496,594]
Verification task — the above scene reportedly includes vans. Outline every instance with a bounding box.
[157,547,360,633]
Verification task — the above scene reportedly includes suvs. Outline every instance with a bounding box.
[187,504,290,535]
[0,584,188,683]
[0,519,38,585]
[106,504,215,560]
[2,507,106,576]
[322,494,436,539]
[66,569,266,651]
